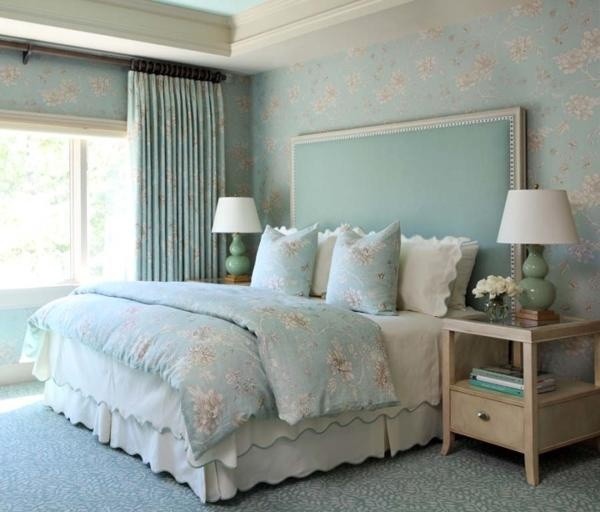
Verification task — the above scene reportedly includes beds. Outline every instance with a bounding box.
[48,105,526,509]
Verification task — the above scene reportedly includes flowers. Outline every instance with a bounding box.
[472,274,523,316]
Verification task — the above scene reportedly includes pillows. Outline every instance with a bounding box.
[249,223,479,318]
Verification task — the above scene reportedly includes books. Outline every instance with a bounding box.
[469,381,557,399]
[469,374,556,390]
[471,363,551,385]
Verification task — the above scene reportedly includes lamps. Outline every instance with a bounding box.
[211,197,263,282]
[496,184,579,321]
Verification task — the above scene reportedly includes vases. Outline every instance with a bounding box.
[484,296,510,321]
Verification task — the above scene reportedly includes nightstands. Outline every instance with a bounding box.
[440,310,600,487]
